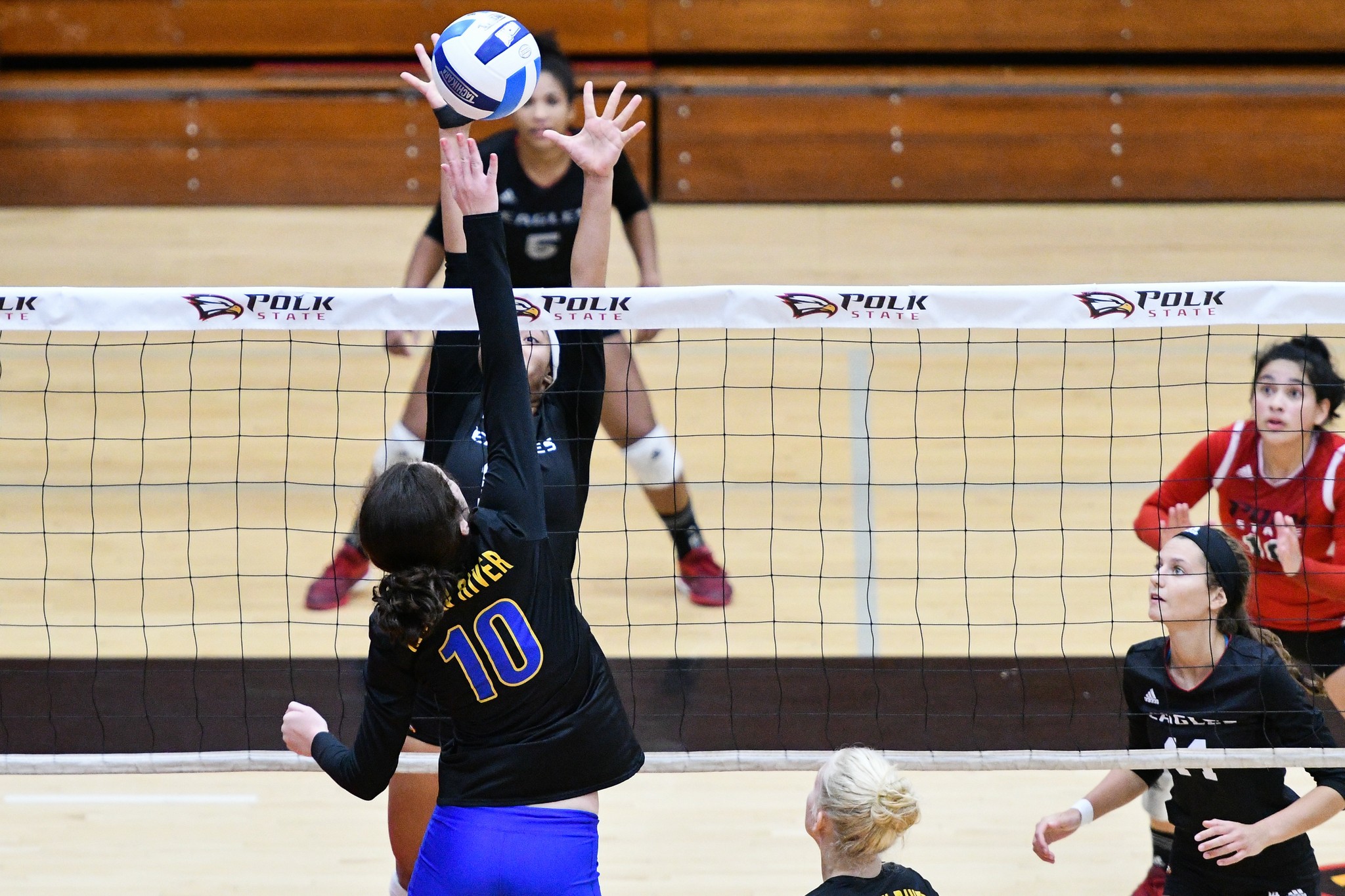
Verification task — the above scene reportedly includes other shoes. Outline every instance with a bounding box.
[1130,864,1166,896]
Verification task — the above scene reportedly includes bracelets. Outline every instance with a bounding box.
[1069,799,1094,830]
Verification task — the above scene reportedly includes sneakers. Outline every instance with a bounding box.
[304,543,370,609]
[674,544,735,607]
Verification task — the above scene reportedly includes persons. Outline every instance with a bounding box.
[305,33,732,610]
[1033,525,1345,896]
[387,33,647,895]
[1134,335,1345,719]
[279,135,646,896]
[805,749,937,895]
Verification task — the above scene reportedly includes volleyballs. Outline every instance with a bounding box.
[431,11,541,120]
